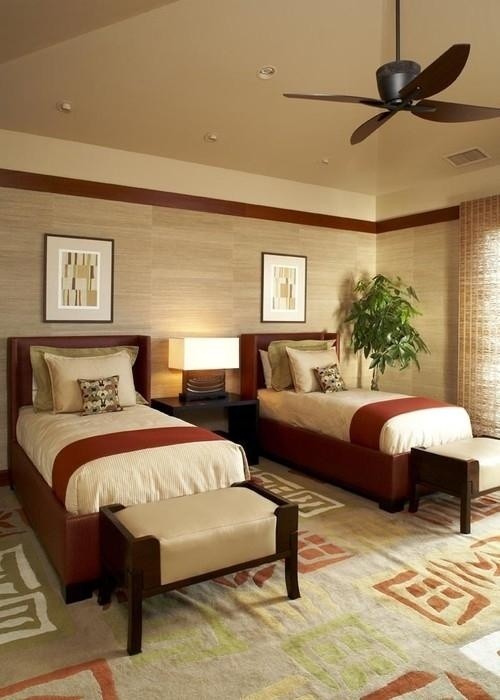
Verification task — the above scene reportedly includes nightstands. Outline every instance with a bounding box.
[152,392,259,465]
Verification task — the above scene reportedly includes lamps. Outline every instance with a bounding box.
[168,336,240,403]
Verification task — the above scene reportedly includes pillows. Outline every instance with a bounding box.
[259,339,348,394]
[30,345,149,417]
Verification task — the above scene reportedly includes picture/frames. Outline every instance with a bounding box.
[42,233,115,323]
[262,252,307,322]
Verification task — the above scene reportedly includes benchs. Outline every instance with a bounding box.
[97,480,300,656]
[409,435,500,534]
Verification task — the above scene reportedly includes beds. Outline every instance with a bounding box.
[7,335,249,604]
[241,334,474,514]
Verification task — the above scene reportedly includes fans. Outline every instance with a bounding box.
[283,1,500,145]
